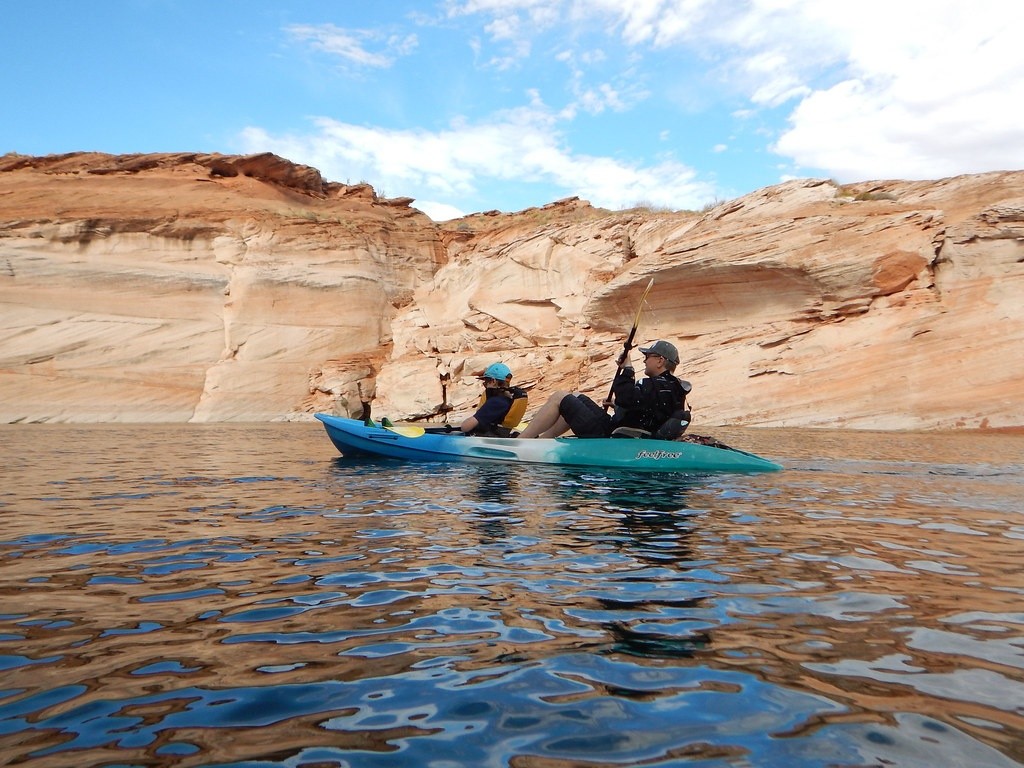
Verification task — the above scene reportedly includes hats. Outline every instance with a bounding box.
[638,341,680,365]
[475,362,513,382]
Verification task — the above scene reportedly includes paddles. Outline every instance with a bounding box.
[383,424,459,439]
[602,277,654,412]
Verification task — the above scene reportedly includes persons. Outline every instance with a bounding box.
[506,340,692,441]
[364,361,513,438]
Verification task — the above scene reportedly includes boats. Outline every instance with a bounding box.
[315,413,784,472]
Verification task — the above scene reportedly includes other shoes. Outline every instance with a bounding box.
[364,417,375,427]
[382,418,393,429]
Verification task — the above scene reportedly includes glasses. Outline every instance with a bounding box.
[646,354,660,360]
[483,379,491,387]
